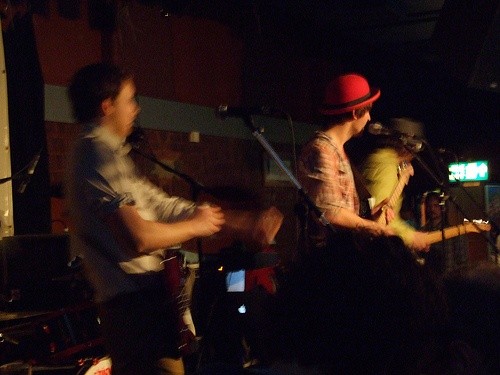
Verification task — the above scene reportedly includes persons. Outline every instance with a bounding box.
[296,73,431,251]
[422,191,467,275]
[67,61,226,375]
[362,117,425,226]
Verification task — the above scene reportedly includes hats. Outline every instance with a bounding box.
[384,117,429,147]
[312,73,381,116]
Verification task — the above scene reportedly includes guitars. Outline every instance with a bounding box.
[370,219,491,285]
[353,161,414,253]
[164,247,198,352]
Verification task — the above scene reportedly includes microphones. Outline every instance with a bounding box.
[409,141,446,155]
[369,123,417,140]
[217,103,283,120]
[17,153,41,193]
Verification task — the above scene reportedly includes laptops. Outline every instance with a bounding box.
[0,233,71,288]
